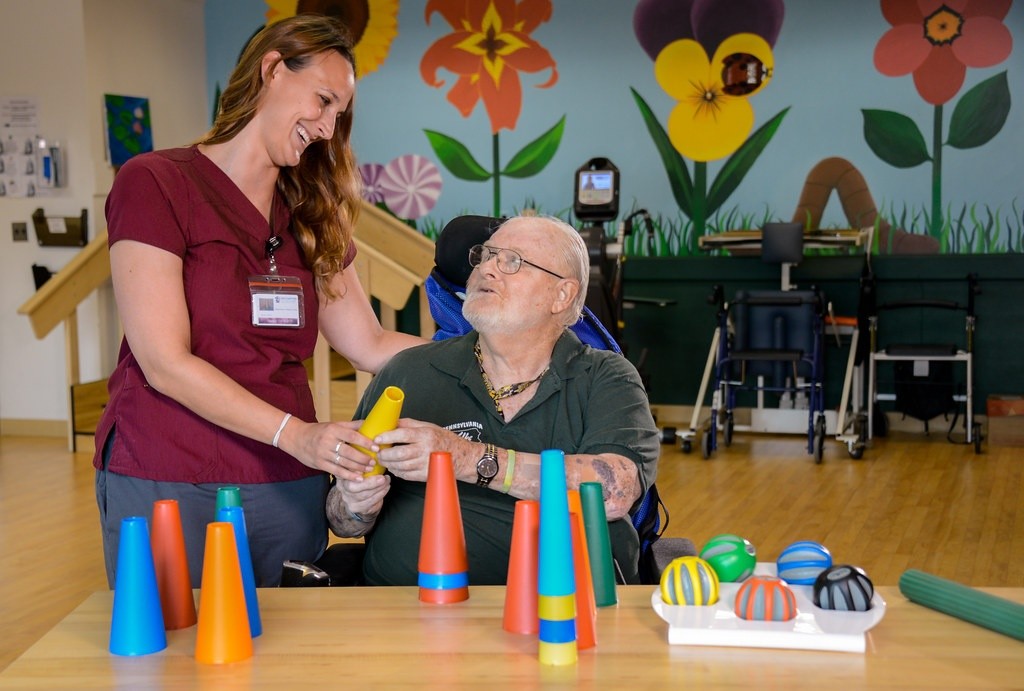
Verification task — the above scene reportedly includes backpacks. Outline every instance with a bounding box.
[423,215,670,567]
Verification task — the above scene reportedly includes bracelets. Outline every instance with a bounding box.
[502,449,515,494]
[350,511,376,524]
[273,413,292,447]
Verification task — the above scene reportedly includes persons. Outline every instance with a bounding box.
[90,17,435,587]
[584,176,594,189]
[324,215,661,585]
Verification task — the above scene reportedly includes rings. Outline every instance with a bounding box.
[334,457,341,465]
[335,441,342,453]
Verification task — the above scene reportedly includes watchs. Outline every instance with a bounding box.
[475,443,500,490]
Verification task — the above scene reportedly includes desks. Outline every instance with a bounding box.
[1,586,1024,691]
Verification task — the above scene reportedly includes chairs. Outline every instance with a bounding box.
[276,215,674,594]
[868,308,987,455]
[702,291,827,465]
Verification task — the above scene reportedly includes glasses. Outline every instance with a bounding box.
[469,244,566,280]
